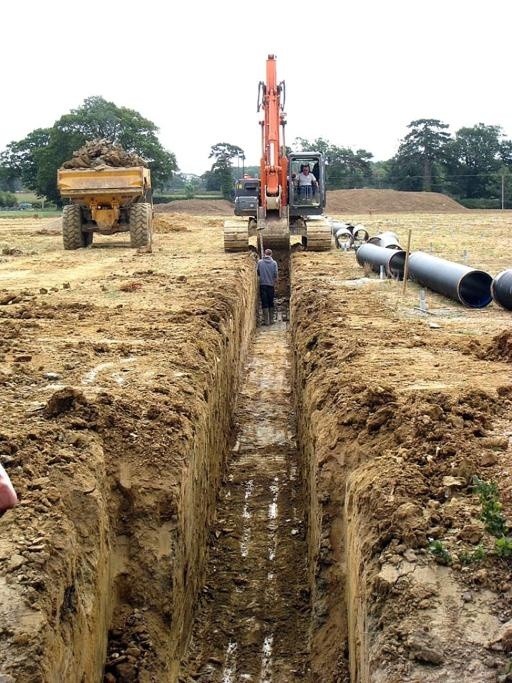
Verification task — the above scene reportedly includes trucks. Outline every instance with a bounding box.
[56,168,153,251]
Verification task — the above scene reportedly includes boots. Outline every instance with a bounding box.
[269,308,274,323]
[263,308,269,325]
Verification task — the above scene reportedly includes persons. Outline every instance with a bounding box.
[255,248,280,325]
[294,163,319,202]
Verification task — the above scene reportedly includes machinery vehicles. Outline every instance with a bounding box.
[222,53,334,253]
